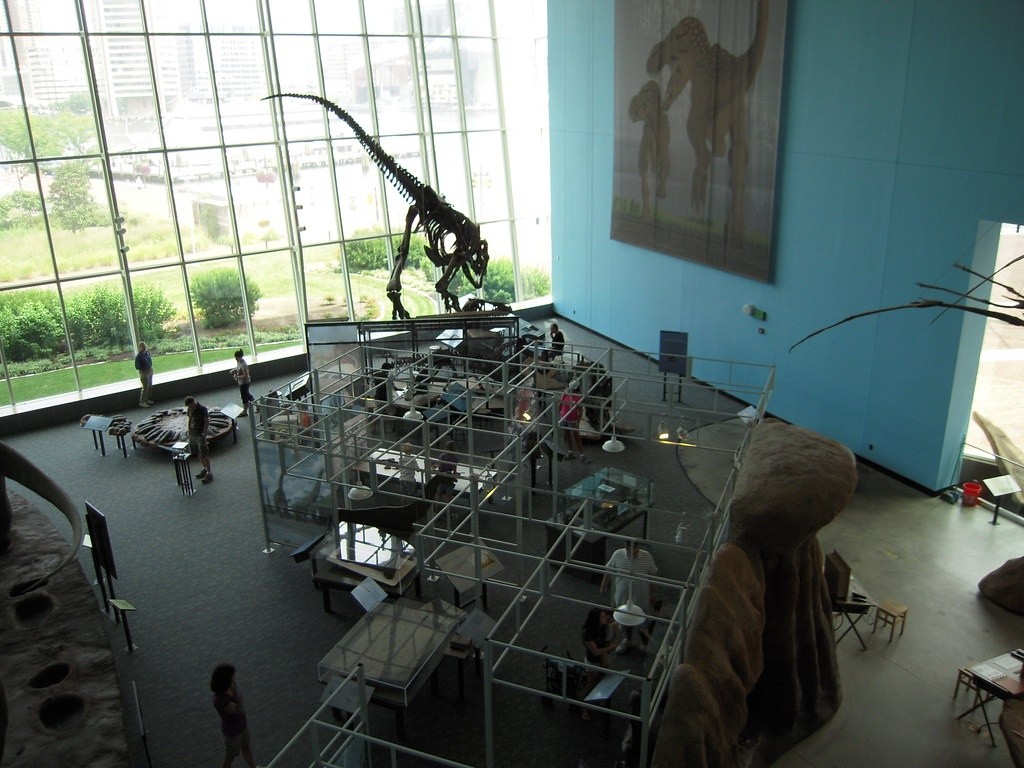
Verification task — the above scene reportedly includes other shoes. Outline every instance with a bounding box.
[564,451,575,460]
[582,711,592,723]
[578,453,591,465]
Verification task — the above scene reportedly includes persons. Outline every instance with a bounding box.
[581,610,622,721]
[210,663,265,768]
[600,538,660,654]
[373,363,404,401]
[230,349,260,418]
[398,443,419,496]
[548,324,565,357]
[134,341,154,408]
[439,441,458,473]
[560,384,587,457]
[184,396,213,484]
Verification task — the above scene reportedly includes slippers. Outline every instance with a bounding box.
[255,406,260,413]
[238,412,248,417]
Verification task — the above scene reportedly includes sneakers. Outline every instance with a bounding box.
[195,468,213,483]
[640,641,648,652]
[139,399,154,408]
[615,639,633,654]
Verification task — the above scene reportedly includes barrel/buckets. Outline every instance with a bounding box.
[962,483,982,507]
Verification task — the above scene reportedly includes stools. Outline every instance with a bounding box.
[953,668,982,708]
[873,597,908,643]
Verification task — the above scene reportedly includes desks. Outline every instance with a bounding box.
[956,648,1024,748]
[830,575,877,650]
[131,406,238,450]
[109,424,131,458]
[262,330,657,768]
